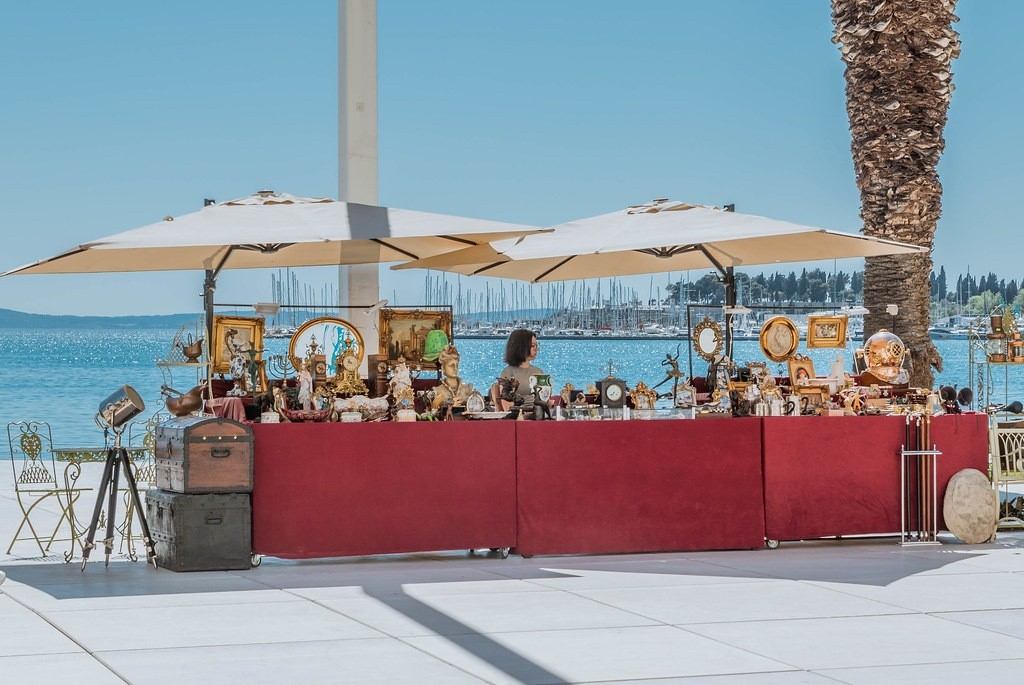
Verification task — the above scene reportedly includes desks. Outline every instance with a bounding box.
[52,447,150,562]
[251,410,988,567]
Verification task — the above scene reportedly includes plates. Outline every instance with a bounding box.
[462,410,512,419]
[881,410,895,415]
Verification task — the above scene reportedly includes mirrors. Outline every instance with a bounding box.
[692,317,724,363]
[288,317,365,379]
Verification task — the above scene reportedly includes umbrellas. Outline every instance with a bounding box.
[385,193,931,362]
[0,187,544,380]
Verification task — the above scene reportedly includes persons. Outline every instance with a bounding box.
[842,372,865,410]
[385,363,413,409]
[499,329,555,413]
[298,362,313,410]
[839,387,858,416]
[706,355,725,399]
[796,371,809,386]
[445,398,454,420]
[759,368,784,401]
[652,343,685,405]
[432,345,485,408]
[529,385,556,420]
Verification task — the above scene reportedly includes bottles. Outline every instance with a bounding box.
[529,373,552,404]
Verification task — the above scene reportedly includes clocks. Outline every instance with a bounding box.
[465,389,485,412]
[594,379,628,408]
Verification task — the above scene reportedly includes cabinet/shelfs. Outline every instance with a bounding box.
[969,305,1024,531]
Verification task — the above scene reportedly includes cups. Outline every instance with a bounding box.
[909,393,927,414]
[260,412,280,424]
[396,409,417,422]
[341,412,362,423]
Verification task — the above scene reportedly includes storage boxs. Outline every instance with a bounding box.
[145,415,253,572]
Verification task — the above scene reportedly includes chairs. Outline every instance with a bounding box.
[6,413,171,557]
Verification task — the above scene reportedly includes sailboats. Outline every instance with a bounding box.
[454,277,689,343]
[260,266,299,338]
[929,264,991,340]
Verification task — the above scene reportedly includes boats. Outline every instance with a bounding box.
[730,319,760,343]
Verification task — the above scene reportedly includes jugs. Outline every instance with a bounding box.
[756,390,809,416]
[927,391,939,415]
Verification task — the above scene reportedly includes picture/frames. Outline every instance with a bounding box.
[671,382,696,406]
[379,309,451,368]
[745,362,766,375]
[807,314,848,348]
[630,382,657,410]
[794,385,830,416]
[211,308,265,373]
[788,353,815,386]
